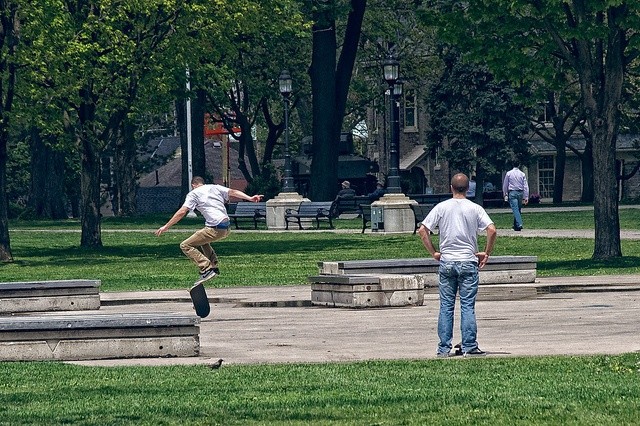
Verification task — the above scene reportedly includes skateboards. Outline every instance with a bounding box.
[187,283,210,318]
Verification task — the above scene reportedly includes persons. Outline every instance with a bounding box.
[419,173,496,358]
[356,180,385,218]
[153,176,265,286]
[503,159,529,231]
[333,181,357,219]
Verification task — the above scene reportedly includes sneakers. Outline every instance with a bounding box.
[199,270,216,280]
[463,348,487,357]
[437,350,449,358]
[210,268,220,277]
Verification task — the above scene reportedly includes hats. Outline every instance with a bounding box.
[377,180,384,186]
[341,181,351,189]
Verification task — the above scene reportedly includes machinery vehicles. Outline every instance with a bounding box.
[291,132,380,195]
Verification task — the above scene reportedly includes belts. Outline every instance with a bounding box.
[509,189,524,192]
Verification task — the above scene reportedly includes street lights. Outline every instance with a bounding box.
[279,67,297,191]
[393,79,404,176]
[380,53,401,193]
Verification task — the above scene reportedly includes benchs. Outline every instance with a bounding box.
[408,192,453,203]
[228,202,267,229]
[284,201,335,229]
[334,194,370,233]
[359,203,371,233]
[410,203,440,235]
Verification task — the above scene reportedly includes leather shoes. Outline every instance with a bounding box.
[205,224,231,230]
[514,226,522,231]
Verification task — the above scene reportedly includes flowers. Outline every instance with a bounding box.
[530,193,541,199]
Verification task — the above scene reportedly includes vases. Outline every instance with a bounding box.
[530,198,540,204]
